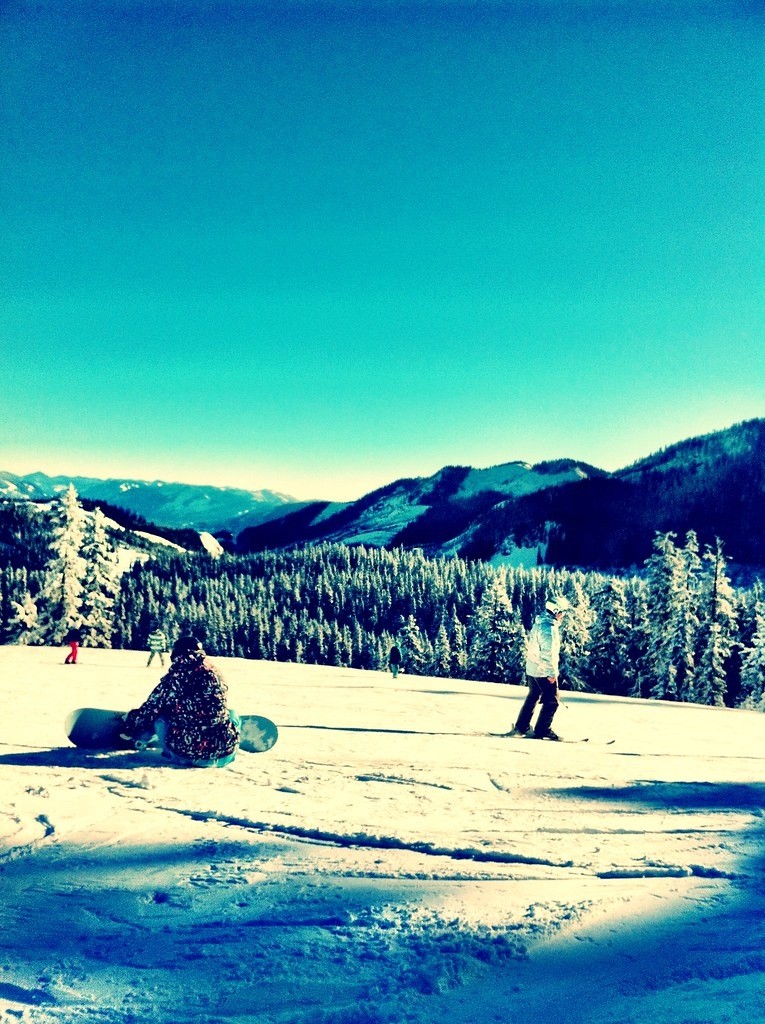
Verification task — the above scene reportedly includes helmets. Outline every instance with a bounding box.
[546,596,568,616]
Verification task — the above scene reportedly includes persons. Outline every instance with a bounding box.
[65,625,79,664]
[146,628,166,667]
[514,597,567,740]
[390,642,402,678]
[120,637,236,767]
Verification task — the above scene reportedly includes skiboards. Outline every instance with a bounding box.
[487,731,616,746]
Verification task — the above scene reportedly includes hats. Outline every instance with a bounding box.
[171,637,202,660]
[394,641,401,647]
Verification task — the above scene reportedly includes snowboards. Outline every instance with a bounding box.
[58,661,83,665]
[65,708,278,753]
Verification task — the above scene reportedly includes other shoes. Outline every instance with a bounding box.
[535,729,562,741]
[65,661,70,664]
[72,661,75,664]
[515,725,535,738]
